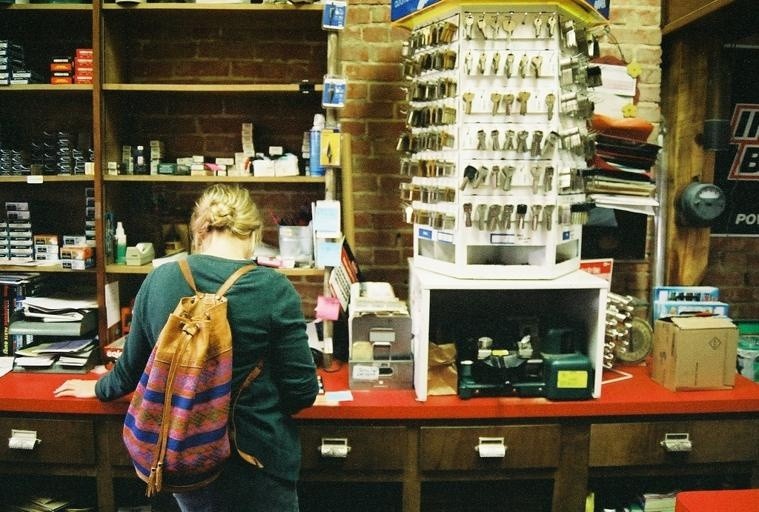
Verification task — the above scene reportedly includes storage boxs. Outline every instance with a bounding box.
[651,316,741,392]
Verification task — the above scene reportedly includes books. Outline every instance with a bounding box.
[0,271,100,376]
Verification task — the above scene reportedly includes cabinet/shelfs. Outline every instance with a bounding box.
[0,0,338,365]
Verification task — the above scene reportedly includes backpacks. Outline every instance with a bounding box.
[122,259,263,493]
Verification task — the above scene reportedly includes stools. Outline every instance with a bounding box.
[407,255,606,403]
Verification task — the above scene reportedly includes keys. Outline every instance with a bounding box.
[395,21,457,230]
[560,20,599,224]
[460,12,557,231]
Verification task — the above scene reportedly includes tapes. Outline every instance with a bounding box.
[136,243,144,252]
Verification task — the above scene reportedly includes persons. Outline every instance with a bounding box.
[52,183,320,510]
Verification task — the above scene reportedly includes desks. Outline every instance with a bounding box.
[0,352,759,511]
